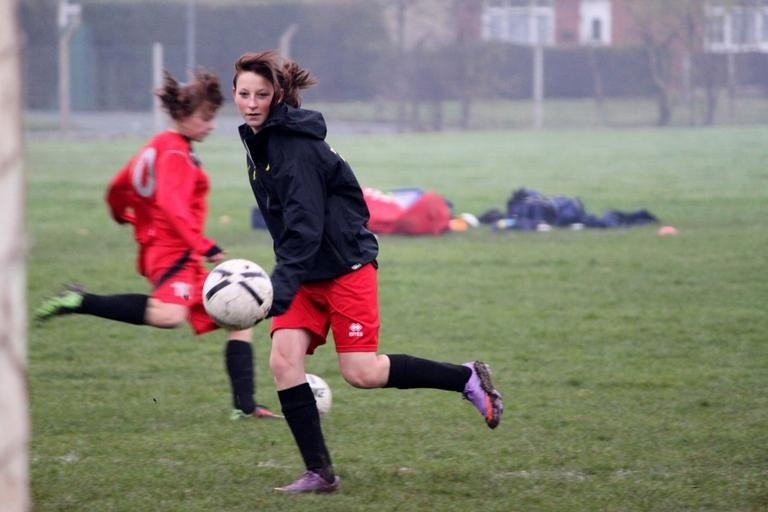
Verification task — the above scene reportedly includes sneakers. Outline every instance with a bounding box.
[32,279,85,322]
[231,406,286,422]
[274,471,342,494]
[461,359,503,429]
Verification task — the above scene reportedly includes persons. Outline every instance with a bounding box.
[28,64,287,425]
[230,50,507,495]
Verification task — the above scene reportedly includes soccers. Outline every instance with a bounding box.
[201,260,274,332]
[304,375,332,418]
